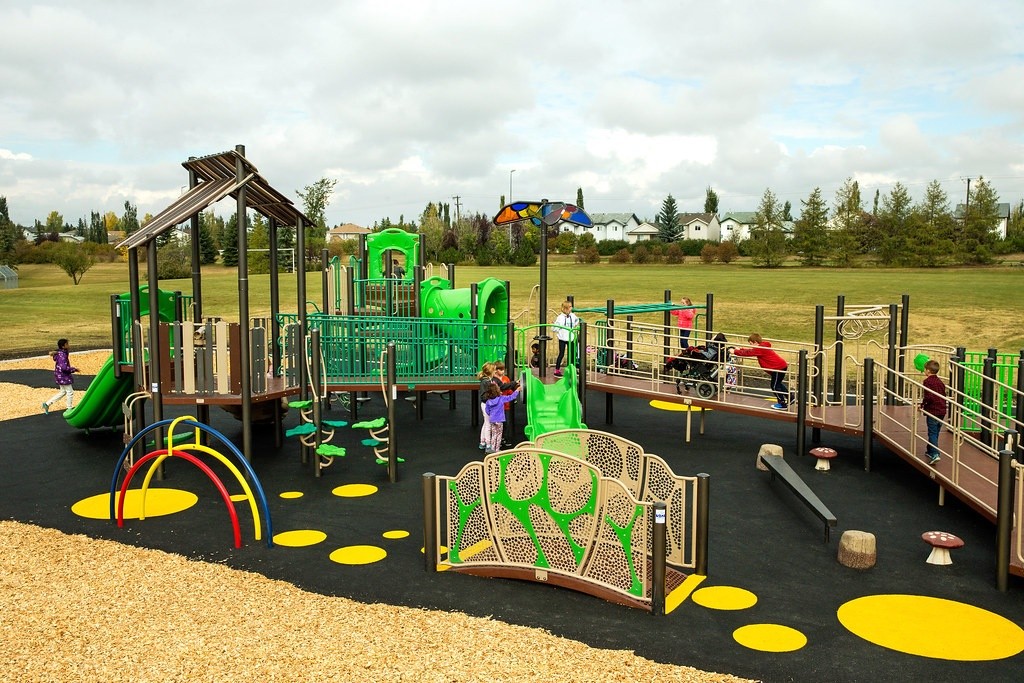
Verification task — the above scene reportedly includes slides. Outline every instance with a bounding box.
[524,369,588,440]
[63,352,128,431]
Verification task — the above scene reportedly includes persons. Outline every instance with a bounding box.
[917,360,947,464]
[530,343,540,368]
[43,339,82,415]
[382,259,405,285]
[477,360,524,455]
[729,333,795,410]
[665,332,728,371]
[670,297,696,348]
[551,301,580,379]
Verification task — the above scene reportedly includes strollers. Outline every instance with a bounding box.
[675,344,741,398]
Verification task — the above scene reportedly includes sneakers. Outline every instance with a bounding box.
[480,444,495,454]
[42,402,50,416]
[771,403,788,410]
[925,451,942,464]
[786,394,796,407]
[554,370,564,379]
